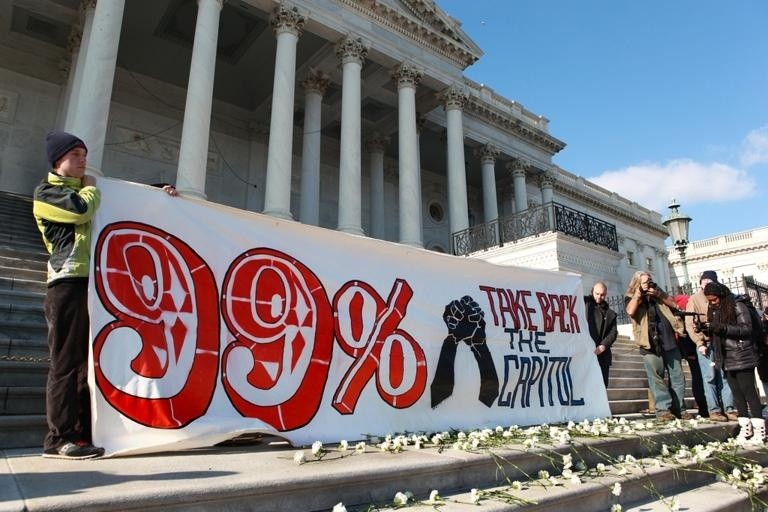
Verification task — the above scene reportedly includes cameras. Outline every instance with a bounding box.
[647,281,657,289]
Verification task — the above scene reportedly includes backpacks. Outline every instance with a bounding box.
[744,302,763,344]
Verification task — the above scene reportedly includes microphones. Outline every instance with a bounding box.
[682,311,705,316]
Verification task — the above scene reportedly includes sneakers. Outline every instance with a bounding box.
[39,439,106,460]
[656,409,738,423]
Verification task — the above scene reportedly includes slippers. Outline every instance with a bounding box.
[731,415,752,445]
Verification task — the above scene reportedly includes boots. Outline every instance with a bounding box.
[740,417,766,451]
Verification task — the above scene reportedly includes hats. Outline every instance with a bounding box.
[698,270,719,285]
[704,281,730,297]
[45,130,89,163]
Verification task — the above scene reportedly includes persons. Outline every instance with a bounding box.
[693,280,768,450]
[670,293,712,419]
[585,281,619,394]
[31,127,179,460]
[624,269,696,422]
[682,269,741,423]
[755,306,768,404]
